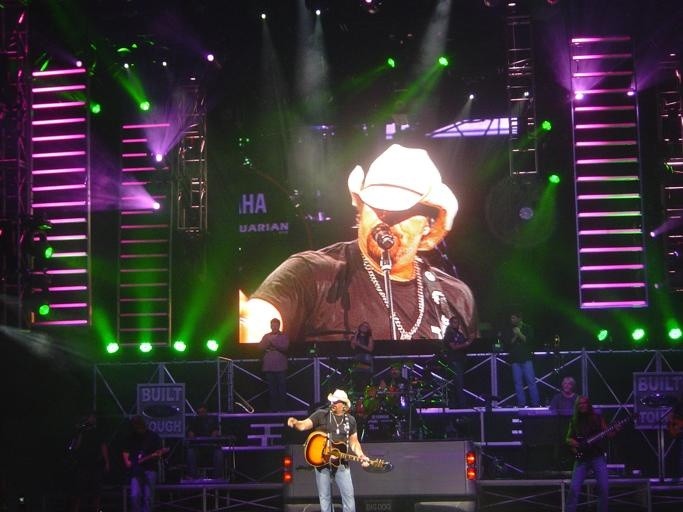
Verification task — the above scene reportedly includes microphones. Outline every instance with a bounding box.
[328,404,334,408]
[370,222,394,250]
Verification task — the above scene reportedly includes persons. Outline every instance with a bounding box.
[287,388,373,512]
[121,414,157,512]
[506,309,540,408]
[256,317,290,412]
[442,315,479,406]
[68,414,113,512]
[191,403,218,436]
[566,394,622,512]
[239,144,481,344]
[547,376,581,410]
[349,321,386,388]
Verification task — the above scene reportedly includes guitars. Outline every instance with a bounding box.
[116,445,171,479]
[567,407,641,460]
[303,431,390,470]
[669,416,682,439]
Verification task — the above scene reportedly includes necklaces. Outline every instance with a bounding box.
[332,413,344,435]
[360,253,425,340]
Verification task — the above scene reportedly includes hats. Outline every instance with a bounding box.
[327,389,351,410]
[347,143,459,251]
